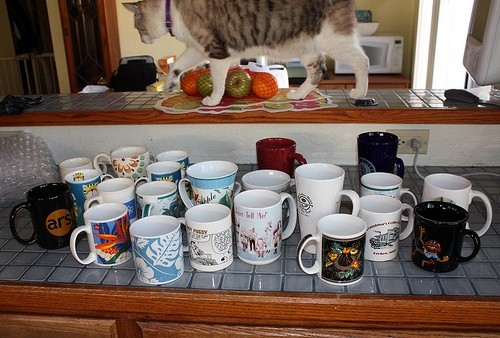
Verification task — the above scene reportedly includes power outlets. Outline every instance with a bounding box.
[387,129,429,154]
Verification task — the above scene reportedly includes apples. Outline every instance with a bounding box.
[198,71,214,97]
[225,70,252,98]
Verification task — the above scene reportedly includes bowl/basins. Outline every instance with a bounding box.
[357,21,379,36]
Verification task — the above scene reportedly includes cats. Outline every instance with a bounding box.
[122,0,370,106]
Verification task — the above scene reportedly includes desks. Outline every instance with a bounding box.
[0,167,499,338]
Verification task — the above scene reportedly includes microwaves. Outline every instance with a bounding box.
[333,32,404,75]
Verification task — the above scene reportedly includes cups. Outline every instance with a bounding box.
[360,172,417,221]
[9,183,78,250]
[59,146,242,286]
[294,163,361,255]
[357,132,405,182]
[242,169,298,224]
[357,195,415,263]
[422,173,492,238]
[256,138,307,179]
[234,190,298,265]
[296,213,367,286]
[412,200,481,274]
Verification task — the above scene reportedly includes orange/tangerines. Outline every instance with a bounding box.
[182,67,278,99]
